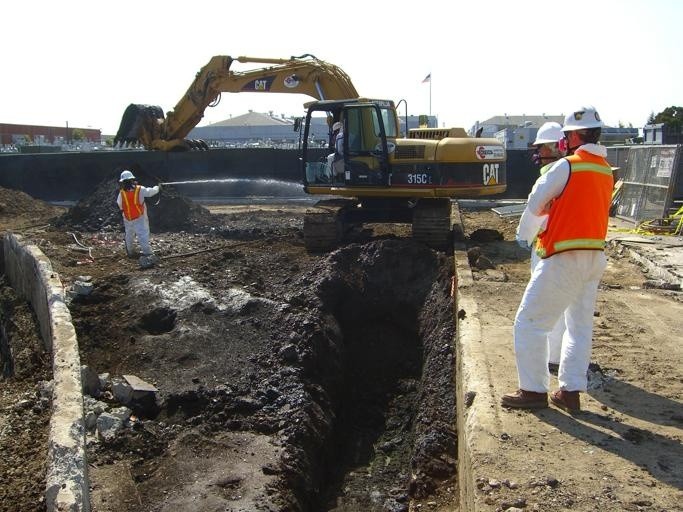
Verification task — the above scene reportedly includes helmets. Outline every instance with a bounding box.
[119,171,136,182]
[532,122,566,146]
[561,105,602,132]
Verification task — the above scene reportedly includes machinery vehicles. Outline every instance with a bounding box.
[112,53,508,250]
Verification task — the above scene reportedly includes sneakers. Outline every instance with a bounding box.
[501,390,548,409]
[550,388,580,414]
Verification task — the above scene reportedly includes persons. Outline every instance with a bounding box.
[499,100,615,413]
[326,121,346,177]
[115,170,164,258]
[530,121,568,373]
